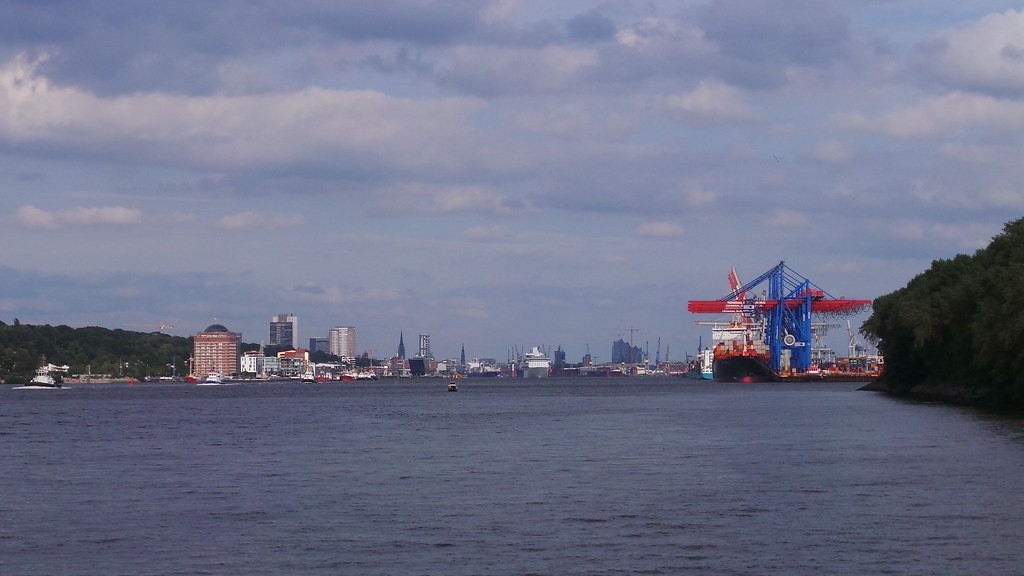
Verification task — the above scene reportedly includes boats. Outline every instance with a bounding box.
[187,375,203,384]
[448,381,458,392]
[206,370,222,383]
[24,354,64,387]
[467,358,502,378]
[316,368,379,382]
[302,370,316,383]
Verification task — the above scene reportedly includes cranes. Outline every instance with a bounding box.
[644,337,669,368]
[129,320,175,332]
[617,326,639,369]
[508,344,523,368]
